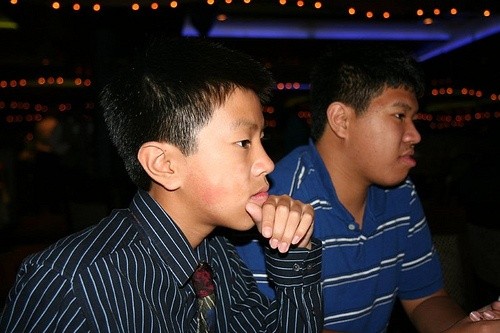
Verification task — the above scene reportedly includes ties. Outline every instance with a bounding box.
[192,262,216,333]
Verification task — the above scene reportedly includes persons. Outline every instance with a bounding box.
[232,51,500,333]
[0,36,324,333]
